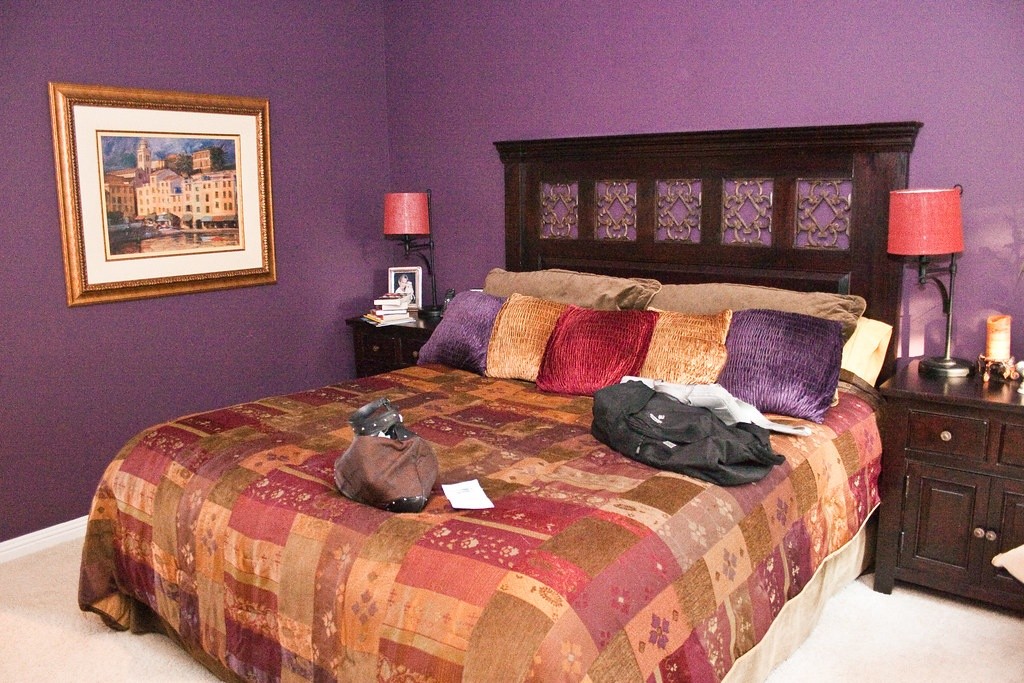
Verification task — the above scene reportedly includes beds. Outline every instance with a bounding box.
[78,120,924,683]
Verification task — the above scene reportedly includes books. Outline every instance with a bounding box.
[361,292,416,327]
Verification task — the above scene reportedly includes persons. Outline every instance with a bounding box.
[395,275,415,303]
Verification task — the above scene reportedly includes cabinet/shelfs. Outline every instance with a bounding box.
[874,359,1024,616]
[346,311,443,378]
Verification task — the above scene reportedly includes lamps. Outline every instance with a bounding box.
[886,183,975,377]
[384,188,443,317]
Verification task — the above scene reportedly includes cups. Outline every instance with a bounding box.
[986,315,1011,361]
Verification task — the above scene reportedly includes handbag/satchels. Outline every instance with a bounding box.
[620,375,812,437]
[330,398,440,514]
[590,380,786,486]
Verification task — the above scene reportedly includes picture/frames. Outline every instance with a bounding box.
[49,81,278,308]
[388,266,423,311]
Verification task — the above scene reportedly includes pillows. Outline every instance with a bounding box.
[639,305,732,385]
[483,293,567,383]
[416,290,508,377]
[536,304,659,396]
[483,268,662,311]
[647,282,866,406]
[715,309,843,425]
